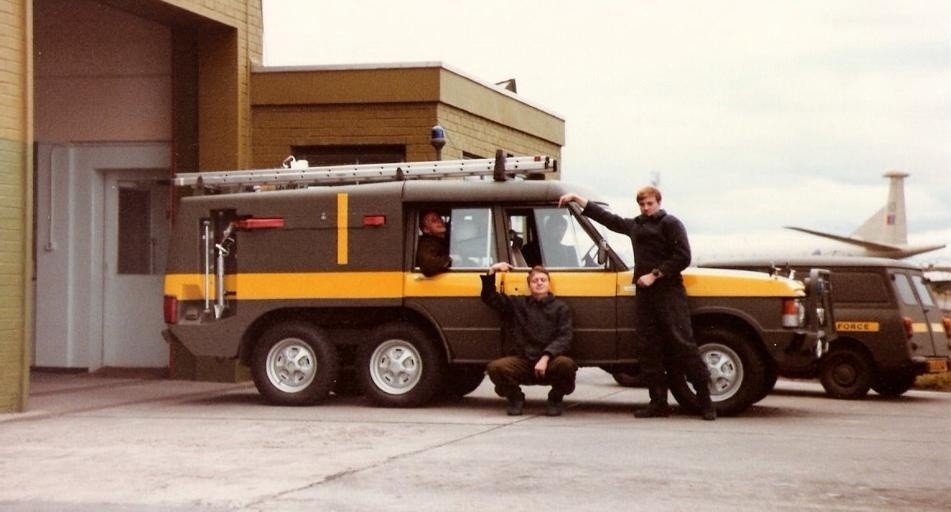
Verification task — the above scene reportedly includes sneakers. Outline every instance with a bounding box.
[633,400,668,417]
[508,398,524,414]
[699,396,717,420]
[547,400,562,415]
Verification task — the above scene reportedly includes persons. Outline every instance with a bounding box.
[416,207,454,276]
[559,185,719,419]
[478,261,579,415]
[521,215,581,268]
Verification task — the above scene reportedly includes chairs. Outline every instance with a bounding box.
[510,245,529,267]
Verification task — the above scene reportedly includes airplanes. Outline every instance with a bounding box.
[557,166,951,270]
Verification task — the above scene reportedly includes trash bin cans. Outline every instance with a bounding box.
[161,328,199,380]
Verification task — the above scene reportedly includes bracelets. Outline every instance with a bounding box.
[652,268,662,278]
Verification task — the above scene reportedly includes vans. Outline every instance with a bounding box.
[610,255,950,398]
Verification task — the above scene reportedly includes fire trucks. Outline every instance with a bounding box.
[159,149,812,415]
